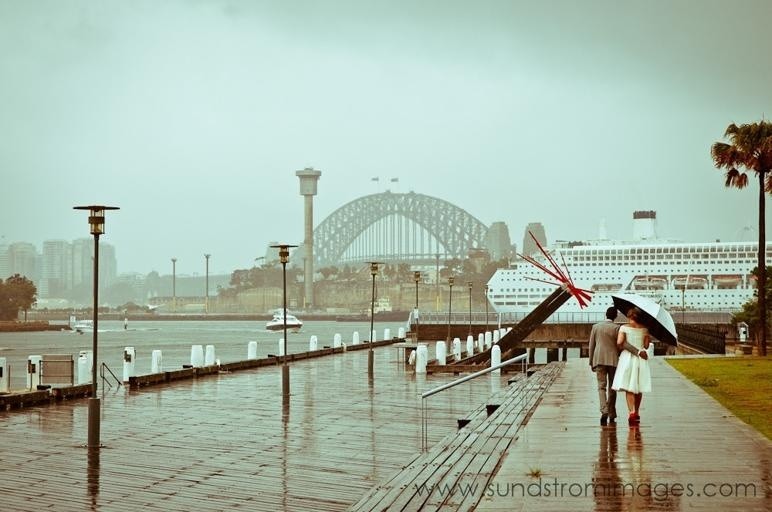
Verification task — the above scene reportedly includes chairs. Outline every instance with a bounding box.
[601,413,617,426]
[629,413,640,422]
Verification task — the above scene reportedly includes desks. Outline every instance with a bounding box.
[204,252,212,312]
[72,205,121,449]
[412,270,491,343]
[171,257,178,313]
[271,244,300,396]
[365,261,386,379]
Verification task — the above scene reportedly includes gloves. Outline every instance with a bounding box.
[266,313,303,331]
[74,318,94,330]
[336,277,411,321]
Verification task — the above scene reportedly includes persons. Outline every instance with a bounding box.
[616,309,650,421]
[588,306,648,425]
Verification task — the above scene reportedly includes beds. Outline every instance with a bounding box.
[611,294,678,346]
